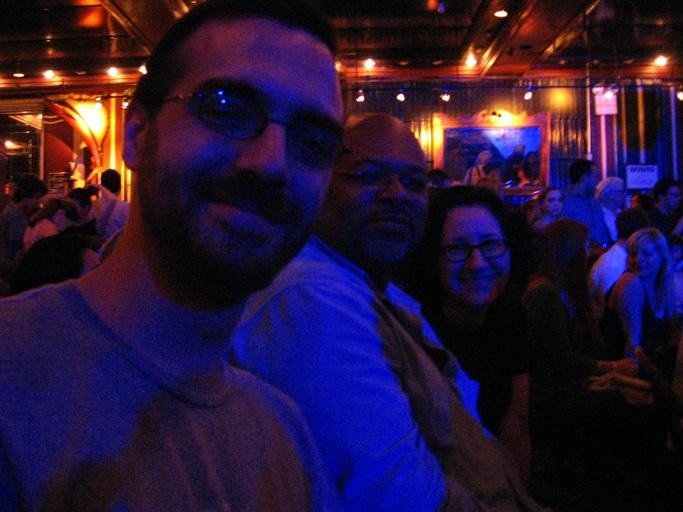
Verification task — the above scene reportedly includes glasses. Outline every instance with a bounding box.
[344,155,436,196]
[155,84,356,169]
[439,239,512,263]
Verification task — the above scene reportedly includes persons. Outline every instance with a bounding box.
[0,1,682,511]
